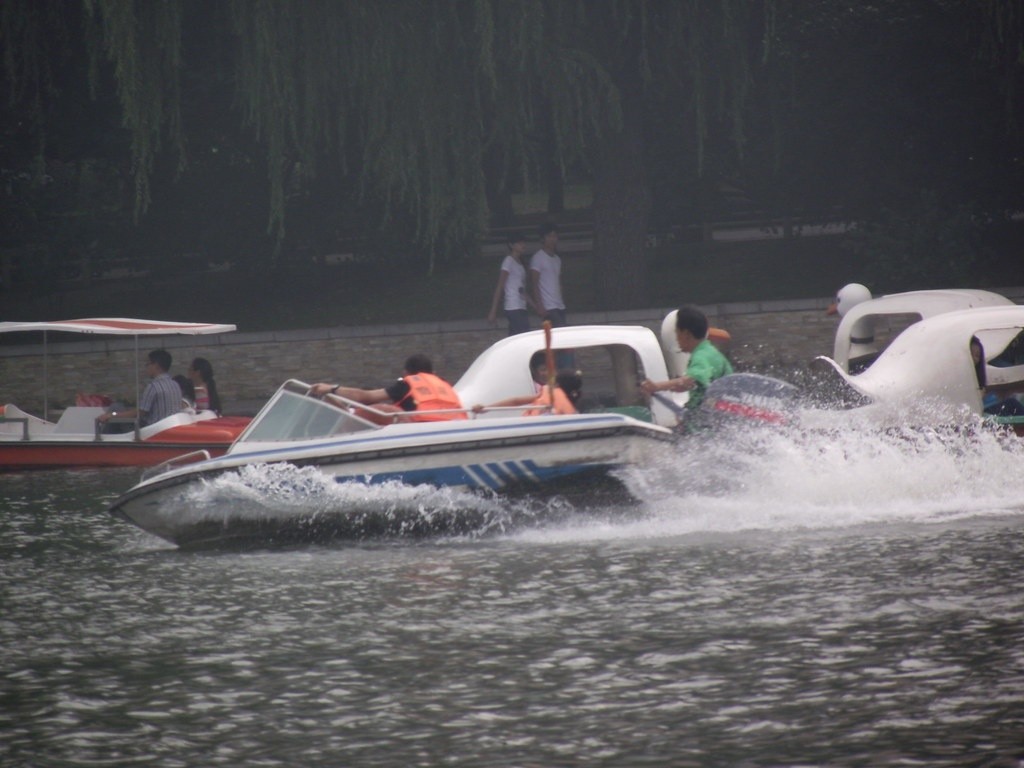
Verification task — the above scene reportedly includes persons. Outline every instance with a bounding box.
[639,305,734,434]
[487,223,568,337]
[98,350,222,434]
[310,354,468,425]
[471,351,583,416]
[970,335,1024,418]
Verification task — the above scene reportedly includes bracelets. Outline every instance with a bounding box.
[111,410,117,416]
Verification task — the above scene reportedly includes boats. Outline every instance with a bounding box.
[103,376,673,548]
[449,279,1024,454]
[0,313,260,468]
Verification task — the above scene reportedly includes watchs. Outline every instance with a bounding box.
[331,384,339,394]
